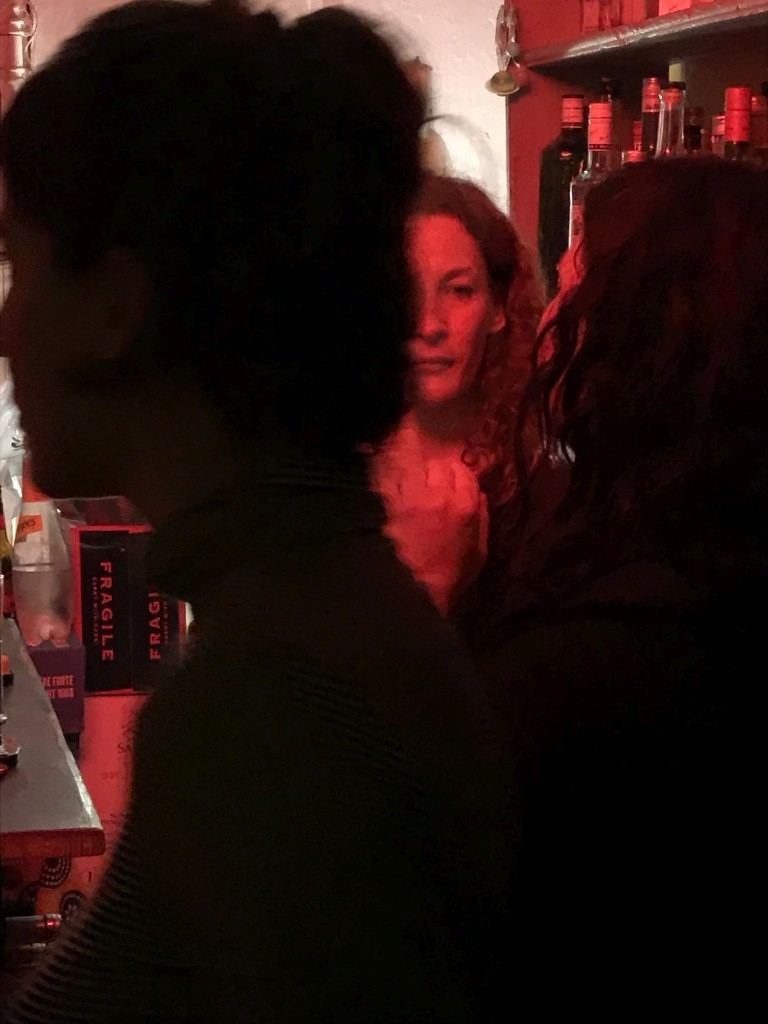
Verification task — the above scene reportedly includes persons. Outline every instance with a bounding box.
[0,1,768,1024]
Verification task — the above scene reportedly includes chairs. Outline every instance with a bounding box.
[33,692,151,929]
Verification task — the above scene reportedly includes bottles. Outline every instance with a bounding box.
[0,439,89,648]
[538,64,768,295]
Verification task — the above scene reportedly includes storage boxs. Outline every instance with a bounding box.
[60,518,133,699]
[130,522,186,693]
[28,630,87,733]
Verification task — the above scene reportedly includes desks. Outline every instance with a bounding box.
[1,617,107,866]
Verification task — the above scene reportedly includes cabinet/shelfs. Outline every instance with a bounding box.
[497,1,768,331]
